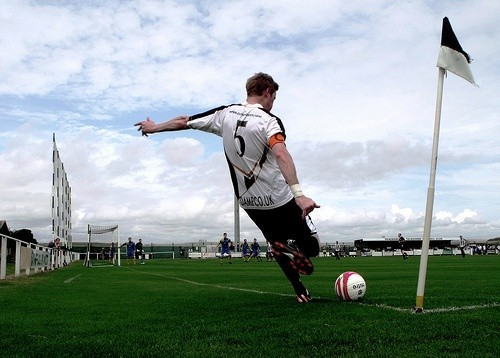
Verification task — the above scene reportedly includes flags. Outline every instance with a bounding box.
[436,16,480,88]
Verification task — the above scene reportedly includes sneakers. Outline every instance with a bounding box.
[271,241,314,275]
[297,291,312,303]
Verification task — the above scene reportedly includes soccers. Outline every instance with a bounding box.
[335,271,366,300]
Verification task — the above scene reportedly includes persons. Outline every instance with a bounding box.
[133,71,321,303]
[101,232,500,264]
[47,238,60,248]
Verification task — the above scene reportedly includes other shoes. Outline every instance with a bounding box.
[141,262,145,264]
